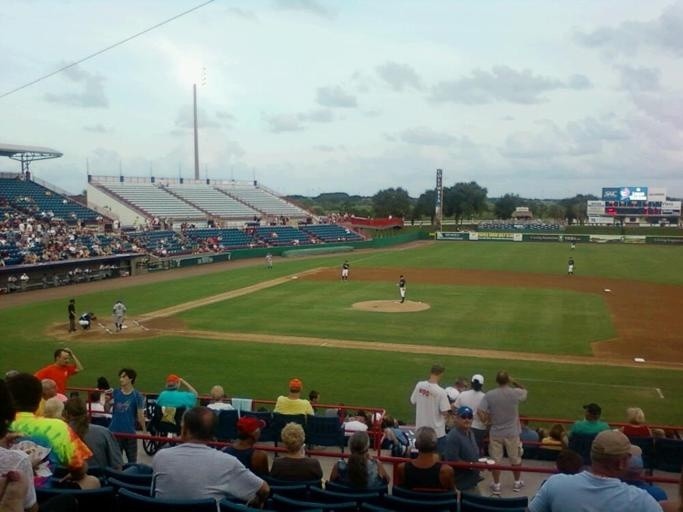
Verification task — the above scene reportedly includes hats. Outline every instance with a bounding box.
[590,430,642,461]
[289,378,304,393]
[236,415,266,437]
[167,374,180,384]
[583,403,601,417]
[458,406,473,418]
[470,373,484,387]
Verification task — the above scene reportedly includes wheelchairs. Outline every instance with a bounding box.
[142,394,187,453]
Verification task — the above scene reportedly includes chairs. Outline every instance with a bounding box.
[33,409,681,510]
[2,178,362,253]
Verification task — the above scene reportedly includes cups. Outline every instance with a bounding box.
[487,459,497,472]
[477,458,487,473]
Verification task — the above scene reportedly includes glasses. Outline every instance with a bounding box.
[461,415,472,420]
